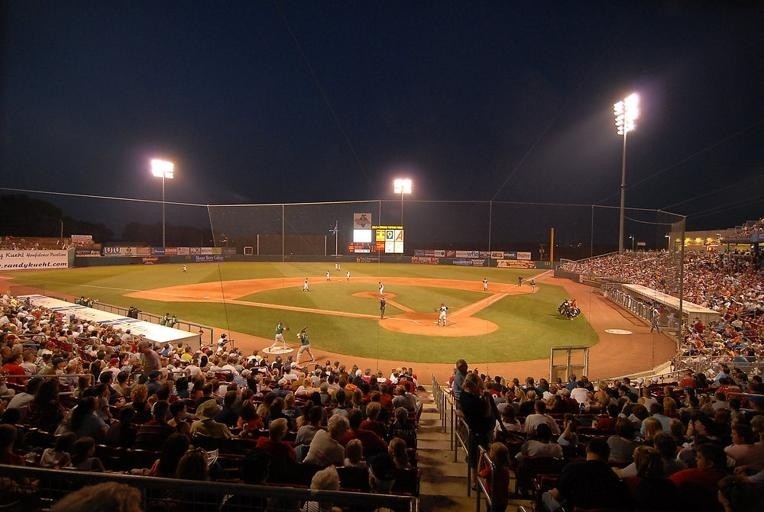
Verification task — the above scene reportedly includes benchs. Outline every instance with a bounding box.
[15,401,297,484]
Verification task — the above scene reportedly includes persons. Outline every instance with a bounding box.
[301,277,309,292]
[378,281,384,296]
[325,270,330,280]
[529,279,535,292]
[559,218,763,284]
[182,262,186,272]
[0,284,424,512]
[482,277,487,291]
[517,275,523,287]
[436,284,764,512]
[344,270,350,280]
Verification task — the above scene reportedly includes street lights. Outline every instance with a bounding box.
[151,154,177,248]
[610,87,645,260]
[393,176,414,225]
